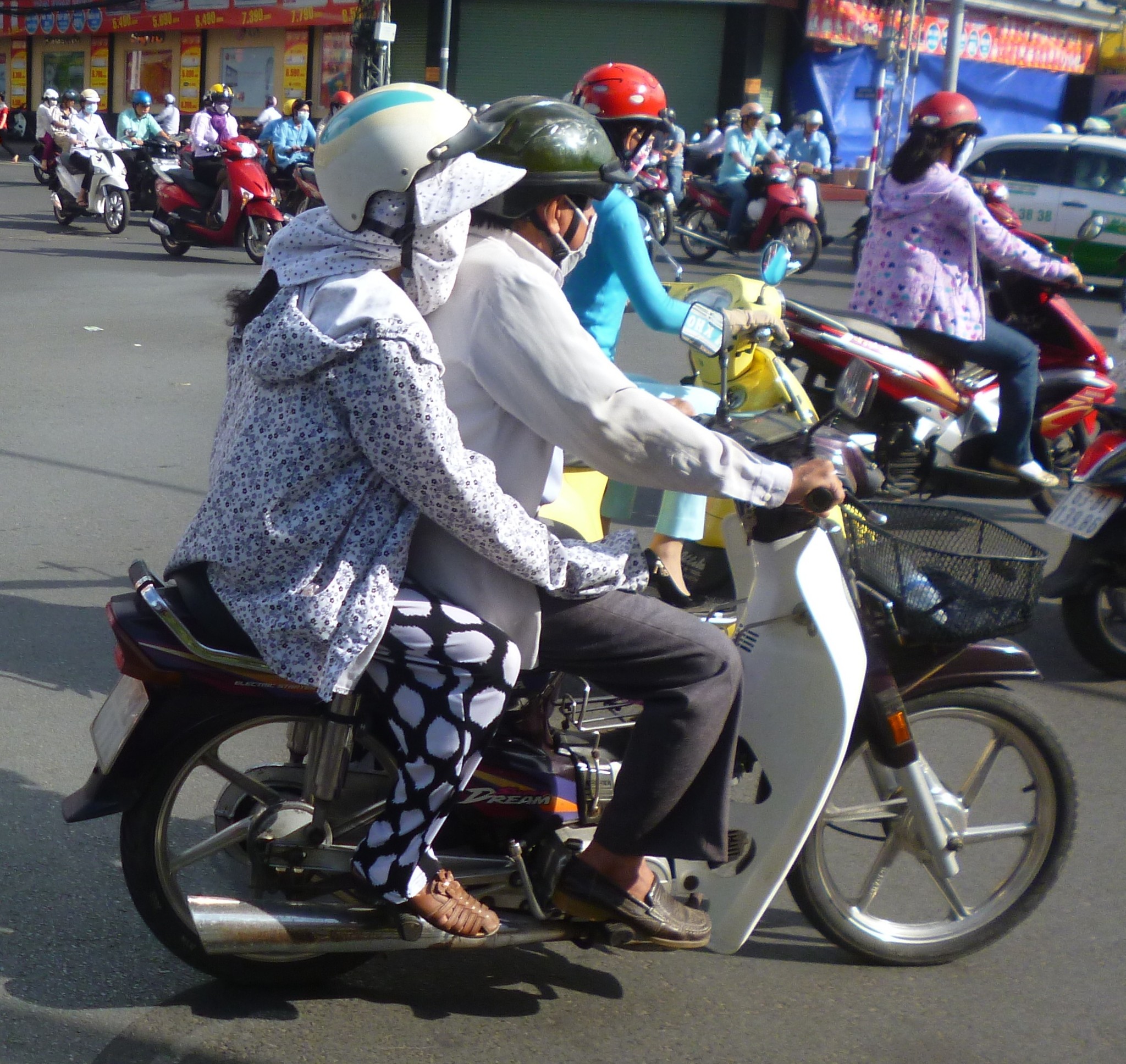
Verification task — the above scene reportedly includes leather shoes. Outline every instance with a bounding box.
[551,853,712,948]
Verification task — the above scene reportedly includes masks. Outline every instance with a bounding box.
[624,134,652,180]
[49,100,58,107]
[214,102,229,115]
[295,109,309,122]
[83,103,98,114]
[544,193,598,277]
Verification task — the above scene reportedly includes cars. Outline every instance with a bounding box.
[954,132,1126,291]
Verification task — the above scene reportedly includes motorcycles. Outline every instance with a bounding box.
[625,131,713,245]
[60,215,1079,979]
[673,158,824,274]
[1038,354,1125,681]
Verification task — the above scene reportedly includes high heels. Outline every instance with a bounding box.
[205,212,221,230]
[644,548,708,608]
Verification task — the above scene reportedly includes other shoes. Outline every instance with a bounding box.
[75,200,87,207]
[989,455,1060,487]
[351,860,502,938]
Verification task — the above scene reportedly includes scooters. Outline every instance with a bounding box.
[757,183,1126,516]
[29,115,327,265]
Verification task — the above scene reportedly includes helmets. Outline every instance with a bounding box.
[291,99,314,115]
[907,91,987,136]
[282,98,296,115]
[202,82,234,99]
[165,94,175,103]
[571,62,667,121]
[267,96,277,106]
[42,88,59,99]
[313,82,478,232]
[63,89,80,100]
[473,95,620,185]
[668,102,822,129]
[333,90,354,105]
[80,88,99,100]
[132,90,152,105]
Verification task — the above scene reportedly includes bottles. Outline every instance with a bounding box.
[876,546,947,622]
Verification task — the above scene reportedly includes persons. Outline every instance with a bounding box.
[420,95,846,951]
[36,82,356,208]
[0,89,19,163]
[848,92,1085,489]
[559,61,794,609]
[160,80,653,949]
